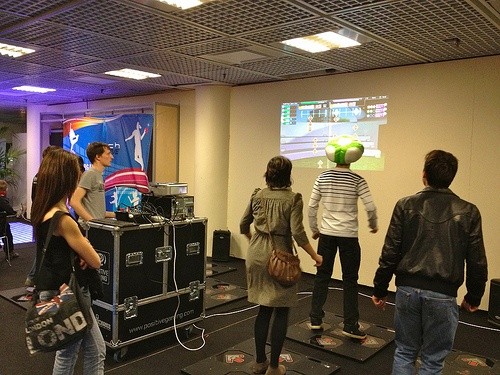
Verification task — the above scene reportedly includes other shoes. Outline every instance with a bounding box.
[265,364,287,375]
[252,358,271,374]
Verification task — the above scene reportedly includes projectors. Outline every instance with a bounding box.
[150,182,189,195]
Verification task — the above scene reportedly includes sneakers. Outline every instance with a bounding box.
[310,321,322,330]
[341,322,368,340]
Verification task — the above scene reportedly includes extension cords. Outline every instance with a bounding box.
[150,216,164,222]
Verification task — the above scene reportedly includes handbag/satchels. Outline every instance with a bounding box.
[24,272,94,357]
[267,248,302,287]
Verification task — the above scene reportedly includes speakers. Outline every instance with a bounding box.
[488,279,500,324]
[212,229,231,261]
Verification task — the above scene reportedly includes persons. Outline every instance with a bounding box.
[239,155,323,375]
[308,134,379,339]
[371,150,488,375]
[0,142,114,375]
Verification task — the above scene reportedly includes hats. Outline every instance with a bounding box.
[325,134,365,165]
